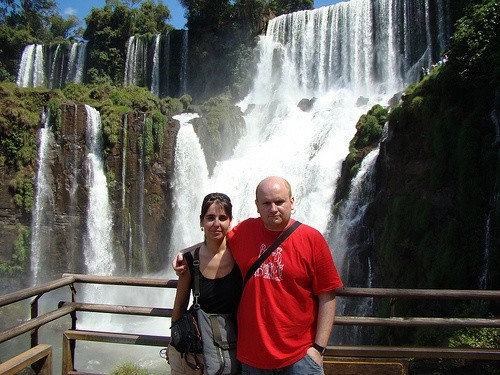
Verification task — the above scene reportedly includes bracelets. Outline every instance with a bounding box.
[313,343,327,356]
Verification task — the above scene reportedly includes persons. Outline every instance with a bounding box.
[172,177,343,375]
[166,193,243,374]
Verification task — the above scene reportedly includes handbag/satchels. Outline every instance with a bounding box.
[197,309,242,375]
[170,306,204,354]
